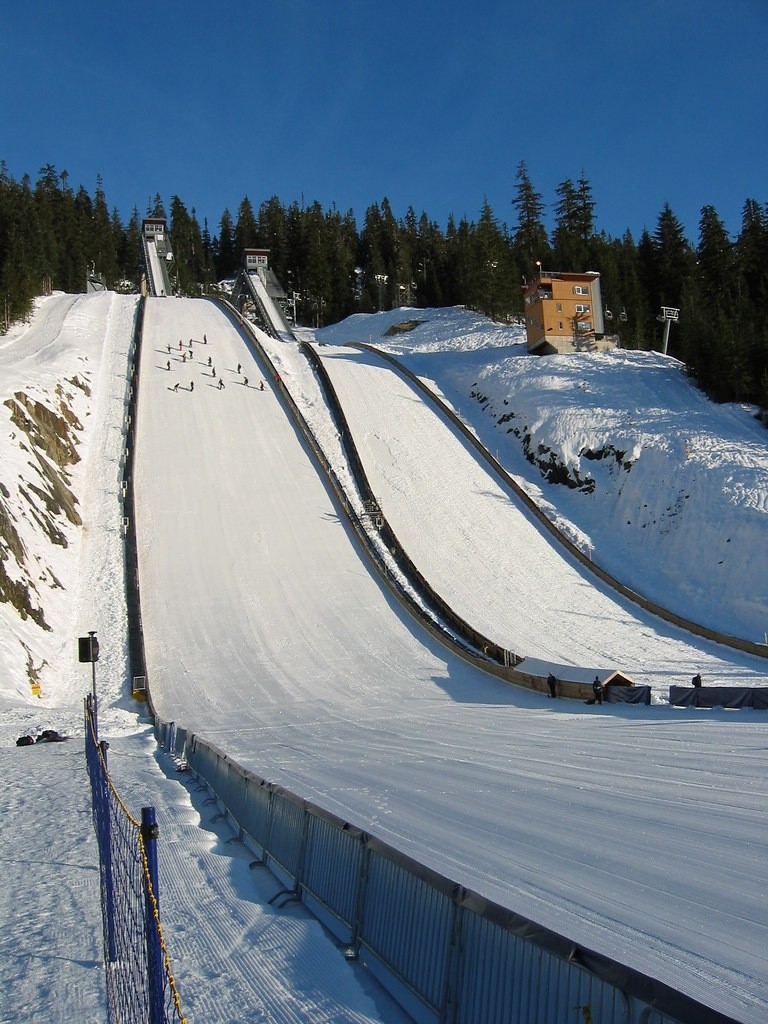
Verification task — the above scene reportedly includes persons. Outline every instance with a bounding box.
[593,676,603,704]
[695,673,702,688]
[167,335,264,393]
[547,672,557,698]
